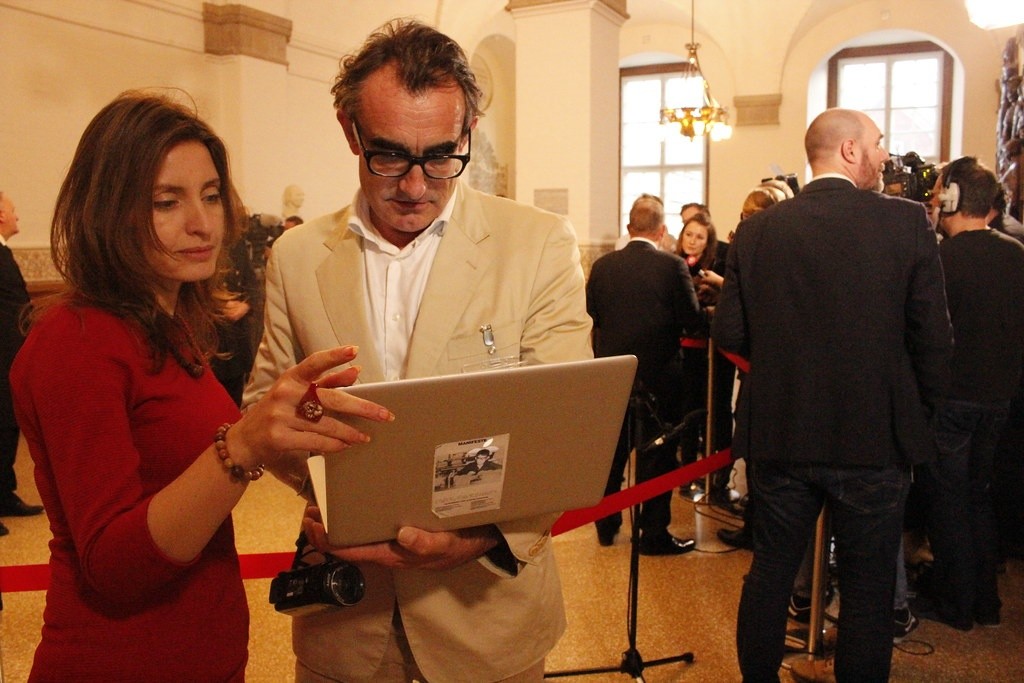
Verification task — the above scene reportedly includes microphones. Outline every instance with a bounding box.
[643,409,708,454]
[681,253,707,277]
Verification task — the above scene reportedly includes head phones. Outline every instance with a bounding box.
[937,158,971,214]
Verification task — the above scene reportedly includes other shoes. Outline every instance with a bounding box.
[974,597,1003,627]
[909,596,973,631]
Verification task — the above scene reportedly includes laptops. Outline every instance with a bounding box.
[309,354,637,546]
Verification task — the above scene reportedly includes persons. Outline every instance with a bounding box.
[239,17,596,683]
[9,86,394,683]
[719,107,957,683]
[0,191,44,537]
[585,156,1024,643]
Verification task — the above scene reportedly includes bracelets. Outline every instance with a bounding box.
[214,422,265,481]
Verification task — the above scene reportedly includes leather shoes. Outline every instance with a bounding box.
[0,523,9,536]
[717,527,744,548]
[639,529,695,555]
[598,529,621,546]
[0,499,45,517]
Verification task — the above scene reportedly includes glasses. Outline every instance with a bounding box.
[350,116,472,180]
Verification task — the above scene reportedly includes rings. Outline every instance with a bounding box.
[299,383,324,421]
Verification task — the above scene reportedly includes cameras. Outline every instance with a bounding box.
[269,558,366,613]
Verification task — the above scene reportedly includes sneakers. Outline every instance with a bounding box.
[893,609,919,644]
[788,594,811,624]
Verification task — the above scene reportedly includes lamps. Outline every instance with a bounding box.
[658,0,732,142]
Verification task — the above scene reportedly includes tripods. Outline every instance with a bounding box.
[543,381,694,683]
